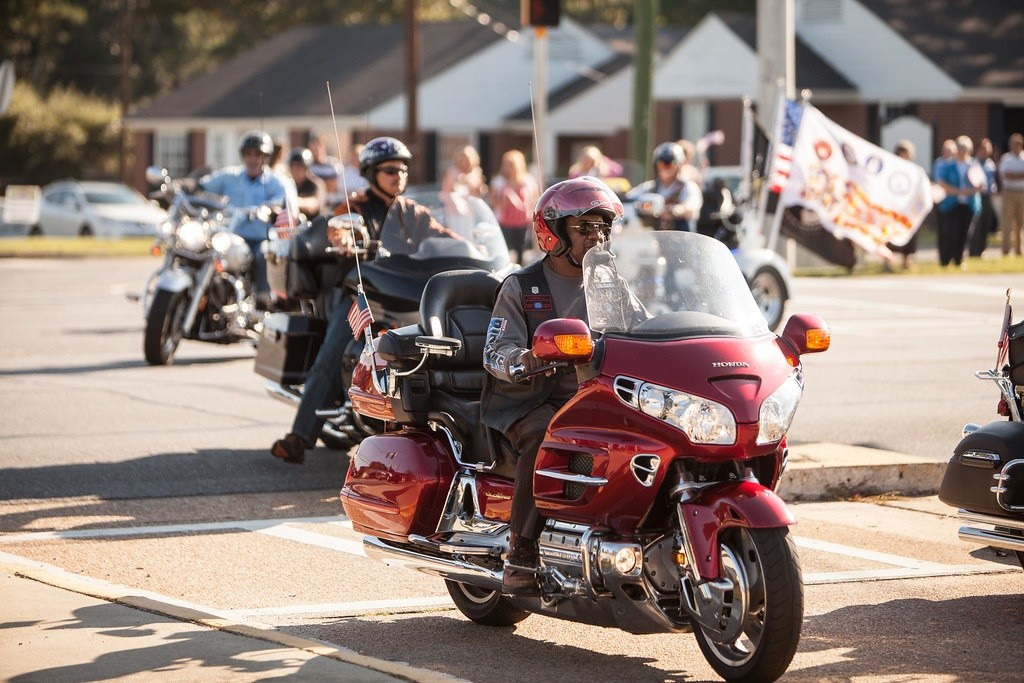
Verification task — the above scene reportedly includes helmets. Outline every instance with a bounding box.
[652,142,685,169]
[238,131,274,156]
[359,138,412,176]
[534,176,623,257]
[291,147,315,166]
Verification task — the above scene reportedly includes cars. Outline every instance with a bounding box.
[29,178,173,238]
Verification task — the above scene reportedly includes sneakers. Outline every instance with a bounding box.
[502,528,540,594]
[272,433,305,464]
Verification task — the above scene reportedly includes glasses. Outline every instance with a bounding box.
[376,165,409,174]
[566,221,609,236]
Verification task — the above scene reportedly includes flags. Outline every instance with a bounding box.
[998,305,1013,366]
[347,293,374,342]
[275,196,299,241]
[799,98,948,258]
[779,95,850,239]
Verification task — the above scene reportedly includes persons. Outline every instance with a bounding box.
[885,133,1024,270]
[175,131,343,310]
[561,146,624,180]
[478,178,801,598]
[440,143,541,268]
[272,136,489,465]
[632,129,741,254]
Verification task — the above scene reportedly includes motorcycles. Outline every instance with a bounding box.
[129,163,293,368]
[937,289,1024,569]
[237,187,515,453]
[349,229,842,682]
